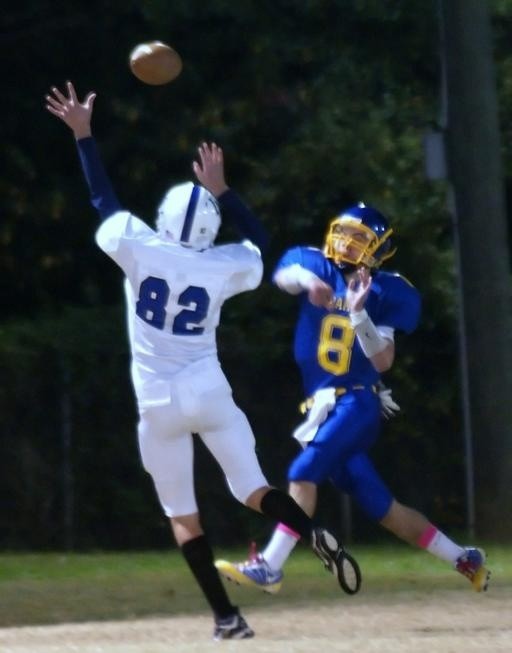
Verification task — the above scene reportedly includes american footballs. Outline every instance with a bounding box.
[130,41,182,85]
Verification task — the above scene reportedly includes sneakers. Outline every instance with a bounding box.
[212,609,255,641]
[456,546,491,592]
[214,553,284,594]
[312,527,362,596]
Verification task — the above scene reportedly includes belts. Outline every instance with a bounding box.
[298,384,377,414]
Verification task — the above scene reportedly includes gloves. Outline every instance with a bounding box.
[380,388,400,420]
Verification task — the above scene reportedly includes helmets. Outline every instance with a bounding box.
[337,203,394,258]
[155,181,222,250]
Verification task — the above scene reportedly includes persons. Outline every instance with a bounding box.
[46,81,362,640]
[214,200,490,596]
